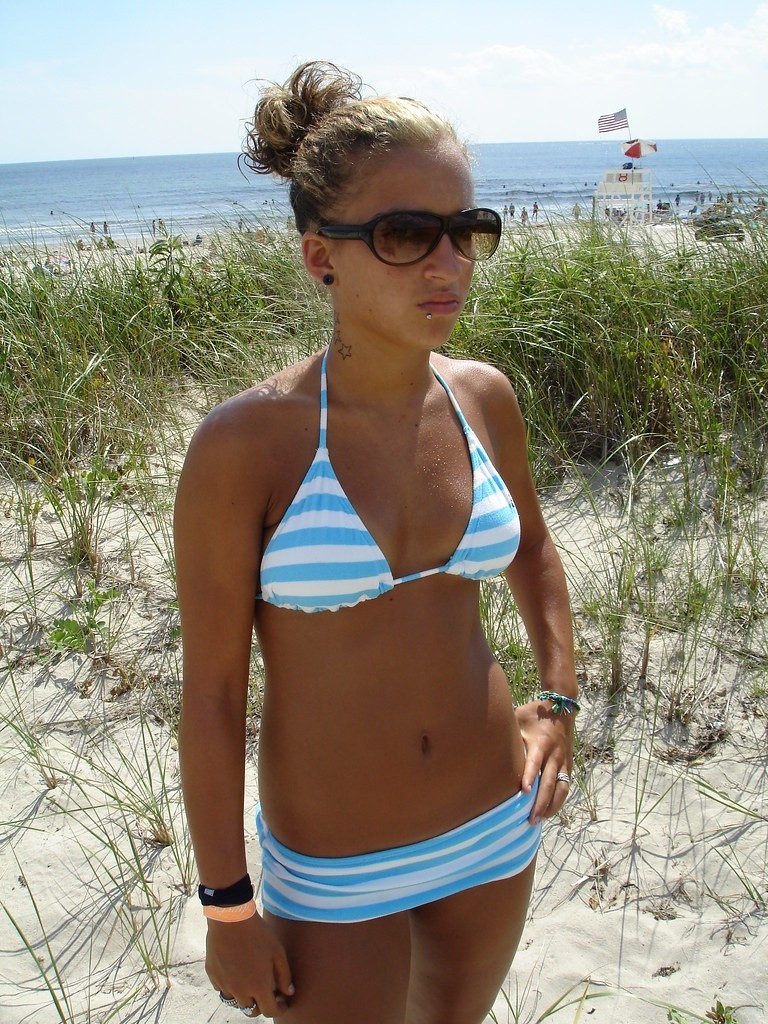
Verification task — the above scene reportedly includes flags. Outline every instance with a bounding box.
[597,108,629,133]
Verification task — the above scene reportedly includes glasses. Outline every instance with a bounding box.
[313,205,506,267]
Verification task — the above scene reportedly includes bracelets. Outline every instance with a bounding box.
[198,874,255,922]
[539,689,580,717]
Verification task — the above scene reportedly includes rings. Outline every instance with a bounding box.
[219,991,237,1007]
[557,772,569,784]
[240,1003,256,1015]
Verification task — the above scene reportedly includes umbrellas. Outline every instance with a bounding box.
[621,138,657,167]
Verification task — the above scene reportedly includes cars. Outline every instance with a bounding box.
[695,219,746,241]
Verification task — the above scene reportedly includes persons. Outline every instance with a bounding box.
[656,180,766,215]
[175,58,581,1024]
[0,198,295,269]
[503,181,598,227]
